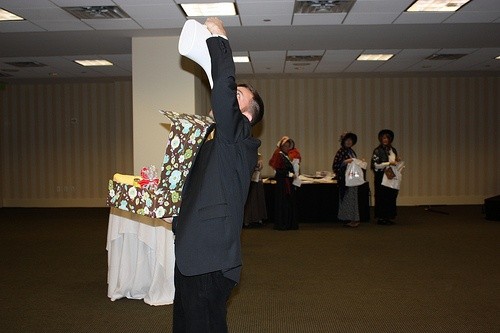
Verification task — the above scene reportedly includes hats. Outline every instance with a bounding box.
[340,131,358,148]
[377,128,395,145]
[275,135,296,151]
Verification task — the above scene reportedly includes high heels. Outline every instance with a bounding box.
[344,221,360,229]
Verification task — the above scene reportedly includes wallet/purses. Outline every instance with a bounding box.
[386,168,395,179]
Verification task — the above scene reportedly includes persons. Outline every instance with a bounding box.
[269,135,301,230]
[243,151,264,228]
[333,130,366,227]
[373,128,400,225]
[172,16,264,332]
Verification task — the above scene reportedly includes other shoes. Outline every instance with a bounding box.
[243,221,250,228]
[376,219,394,226]
[253,220,263,229]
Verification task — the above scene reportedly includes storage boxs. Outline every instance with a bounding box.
[105,109,212,218]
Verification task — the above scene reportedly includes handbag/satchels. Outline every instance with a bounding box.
[381,164,401,190]
[345,157,369,187]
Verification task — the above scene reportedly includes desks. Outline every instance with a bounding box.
[243,176,371,227]
